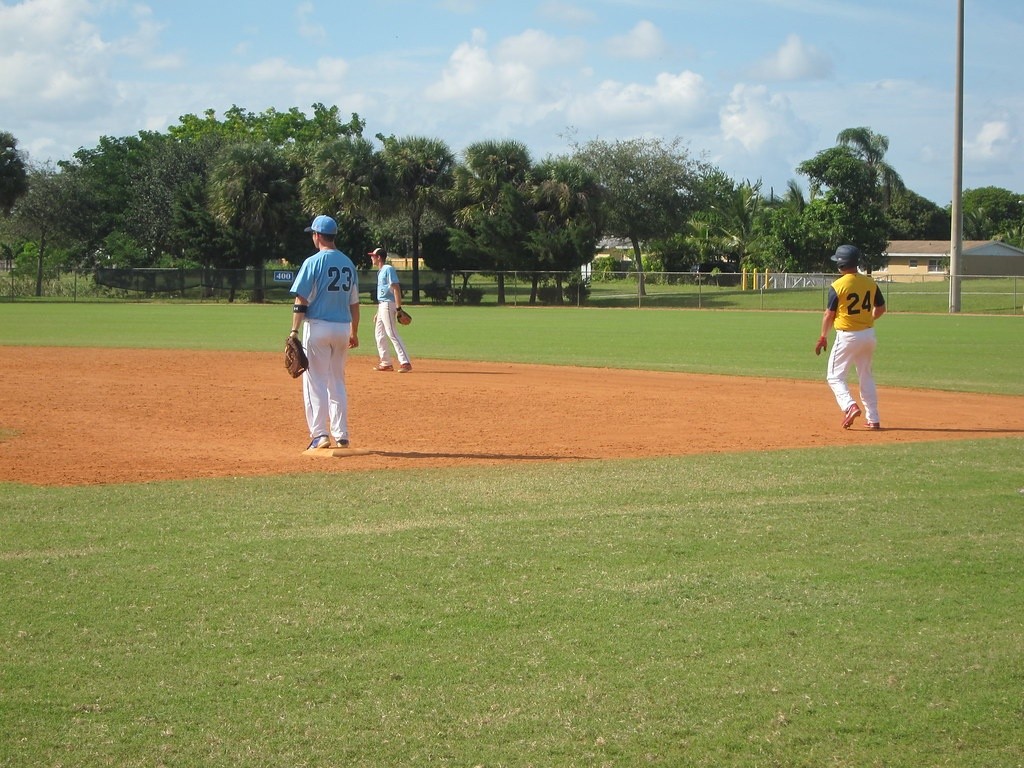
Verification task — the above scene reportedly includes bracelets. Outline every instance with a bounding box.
[290,330,298,333]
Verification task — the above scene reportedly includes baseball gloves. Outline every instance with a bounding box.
[397,309,412,326]
[283,336,309,379]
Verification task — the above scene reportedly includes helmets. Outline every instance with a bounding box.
[830,245,858,268]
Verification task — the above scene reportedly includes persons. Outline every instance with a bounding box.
[816,246,886,428]
[368,248,412,374]
[284,215,360,450]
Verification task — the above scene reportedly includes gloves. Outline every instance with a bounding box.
[815,336,827,355]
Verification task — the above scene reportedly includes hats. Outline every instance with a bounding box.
[304,215,338,234]
[368,248,387,262]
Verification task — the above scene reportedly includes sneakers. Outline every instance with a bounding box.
[864,423,879,428]
[397,364,412,373]
[842,403,861,429]
[337,440,348,448]
[374,365,394,371]
[306,435,331,450]
